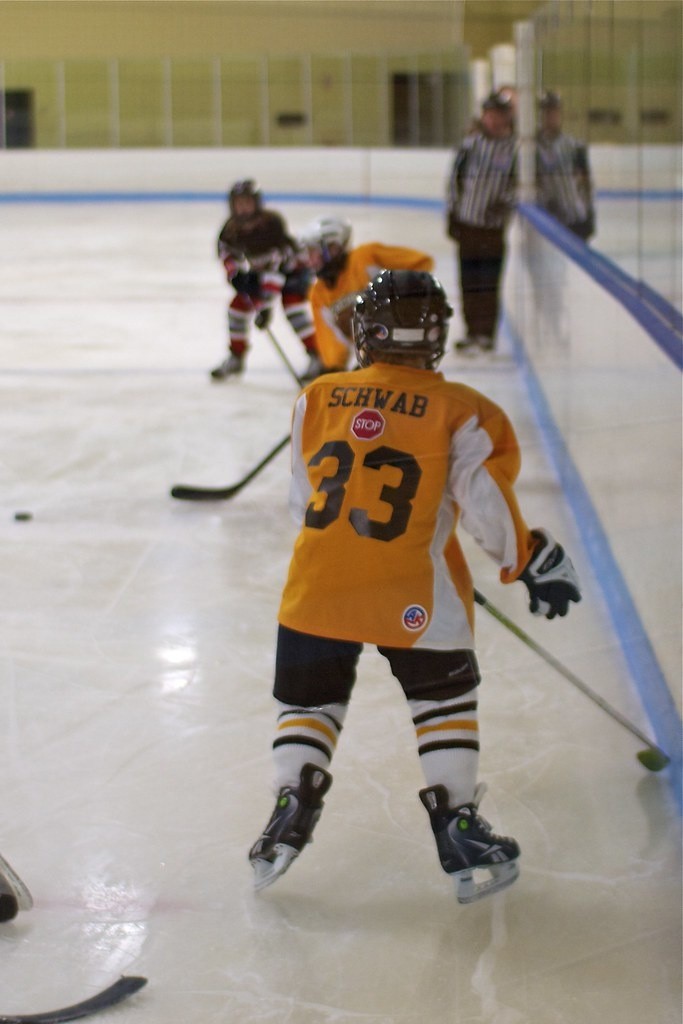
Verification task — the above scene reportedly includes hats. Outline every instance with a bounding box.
[479,92,511,114]
[535,89,565,112]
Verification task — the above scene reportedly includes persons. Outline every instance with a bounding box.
[445,87,521,353]
[0,857,33,922]
[535,89,595,242]
[302,216,437,371]
[212,179,326,381]
[245,268,584,901]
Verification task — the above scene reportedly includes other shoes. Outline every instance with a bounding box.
[210,356,240,380]
[454,336,472,347]
[478,333,496,351]
[302,358,325,381]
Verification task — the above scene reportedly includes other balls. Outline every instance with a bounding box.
[13,510,32,521]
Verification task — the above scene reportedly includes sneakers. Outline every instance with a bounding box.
[418,784,521,903]
[247,763,332,891]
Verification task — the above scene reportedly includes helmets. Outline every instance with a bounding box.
[301,215,352,246]
[355,270,450,361]
[229,179,258,198]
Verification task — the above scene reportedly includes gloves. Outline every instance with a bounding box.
[519,527,581,620]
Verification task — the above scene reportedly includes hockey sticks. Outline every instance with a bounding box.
[170,434,291,501]
[245,295,305,392]
[473,589,672,773]
